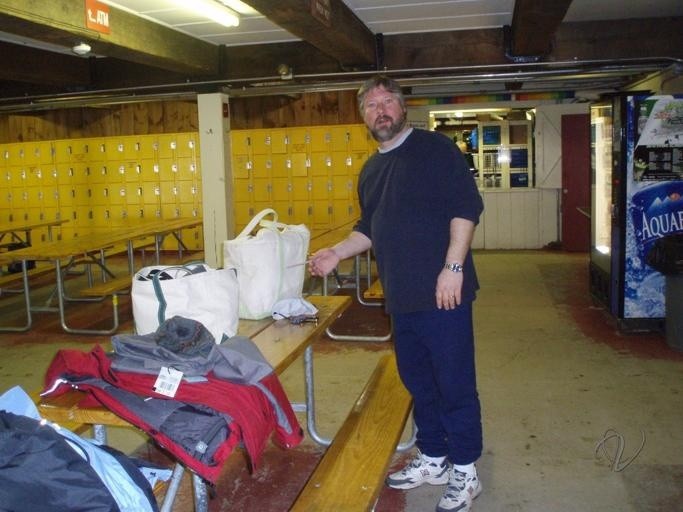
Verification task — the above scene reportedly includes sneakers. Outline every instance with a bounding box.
[436,464,483,512]
[384,450,450,490]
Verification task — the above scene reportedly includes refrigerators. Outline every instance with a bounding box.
[585,95,683,321]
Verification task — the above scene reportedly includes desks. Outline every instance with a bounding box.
[33,293,353,512]
[1,219,204,336]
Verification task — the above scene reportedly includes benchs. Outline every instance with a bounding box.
[288,343,429,512]
[0,238,204,333]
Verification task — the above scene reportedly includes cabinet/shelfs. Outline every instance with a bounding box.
[2,101,202,252]
[229,121,384,277]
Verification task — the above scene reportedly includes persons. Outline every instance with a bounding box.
[306,75,484,512]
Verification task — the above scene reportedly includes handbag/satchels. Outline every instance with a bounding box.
[223,209,310,320]
[131,260,239,346]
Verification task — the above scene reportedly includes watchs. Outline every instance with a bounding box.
[444,263,463,273]
